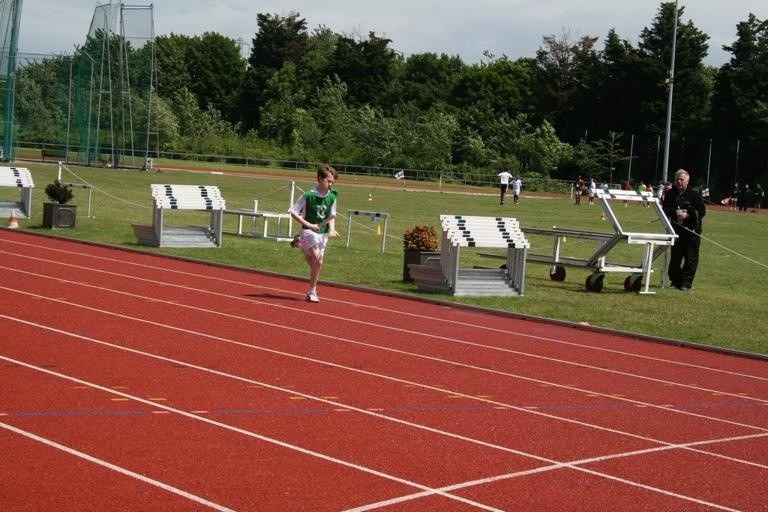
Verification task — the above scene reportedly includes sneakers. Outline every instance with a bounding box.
[668,285,688,292]
[306,291,320,302]
[290,235,300,248]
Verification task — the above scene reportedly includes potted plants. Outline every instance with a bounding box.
[42,178,77,230]
[403,224,438,284]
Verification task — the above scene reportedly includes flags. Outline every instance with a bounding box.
[393,170,405,182]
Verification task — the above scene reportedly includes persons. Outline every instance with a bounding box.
[637,180,647,207]
[750,183,765,214]
[728,181,741,212]
[286,163,342,305]
[661,168,706,293]
[585,176,597,206]
[620,180,632,208]
[665,179,673,189]
[737,184,753,212]
[644,183,654,208]
[599,180,609,190]
[571,175,587,206]
[508,174,523,205]
[652,181,666,201]
[660,182,672,203]
[497,167,516,205]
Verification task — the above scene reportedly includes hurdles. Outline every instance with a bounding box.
[587,188,679,241]
[0,166,34,219]
[344,208,389,250]
[151,184,226,248]
[440,214,530,298]
